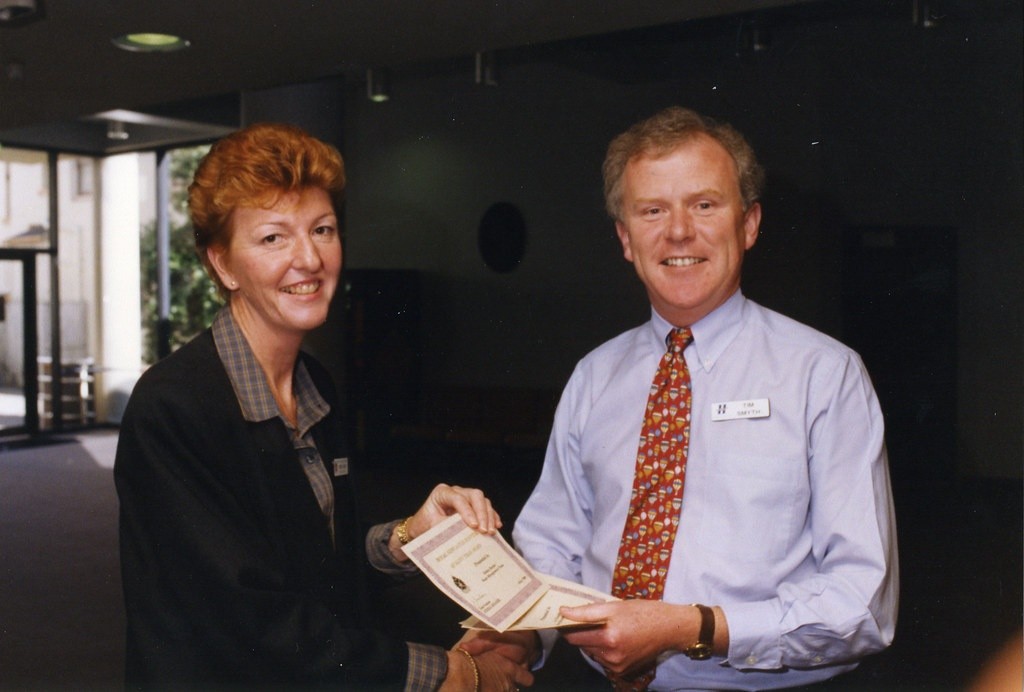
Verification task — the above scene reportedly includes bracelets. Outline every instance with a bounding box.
[396,514,416,547]
[448,646,481,692]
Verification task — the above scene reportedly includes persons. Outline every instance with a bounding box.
[111,123,537,692]
[455,105,904,692]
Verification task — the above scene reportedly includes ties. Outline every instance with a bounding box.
[602,328,695,692]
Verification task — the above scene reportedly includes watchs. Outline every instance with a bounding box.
[685,601,716,661]
